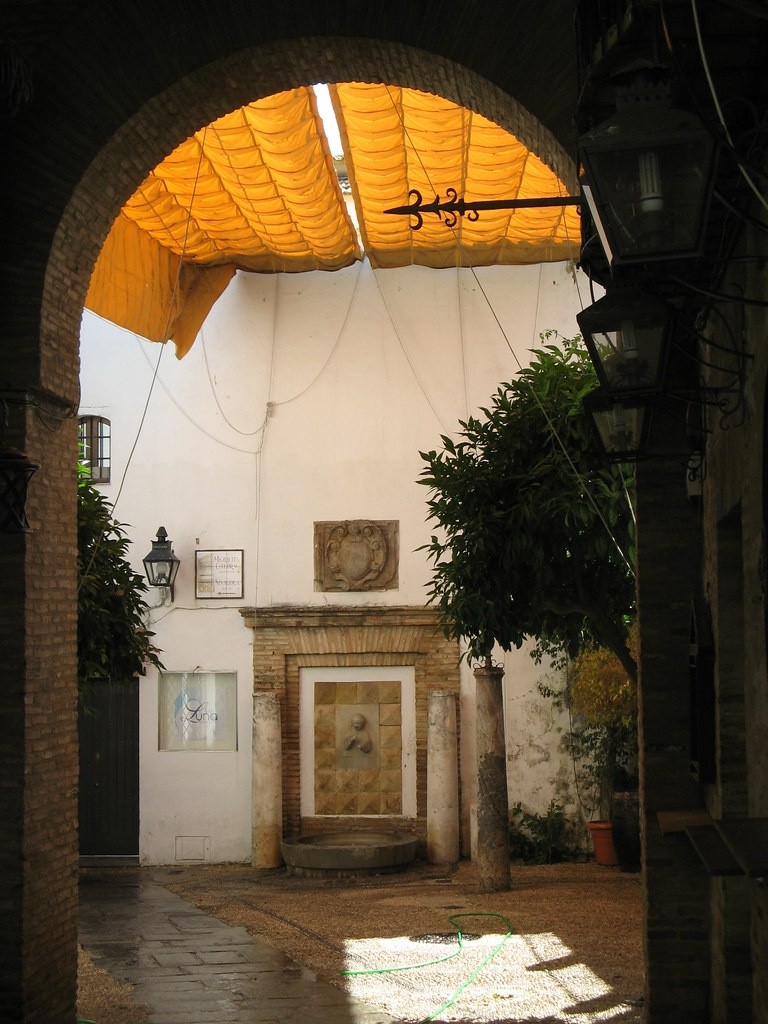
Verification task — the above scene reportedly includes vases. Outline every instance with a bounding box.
[609,790,641,873]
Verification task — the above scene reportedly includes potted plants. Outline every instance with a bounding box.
[536,617,639,866]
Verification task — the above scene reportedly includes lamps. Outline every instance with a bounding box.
[142,526,181,603]
